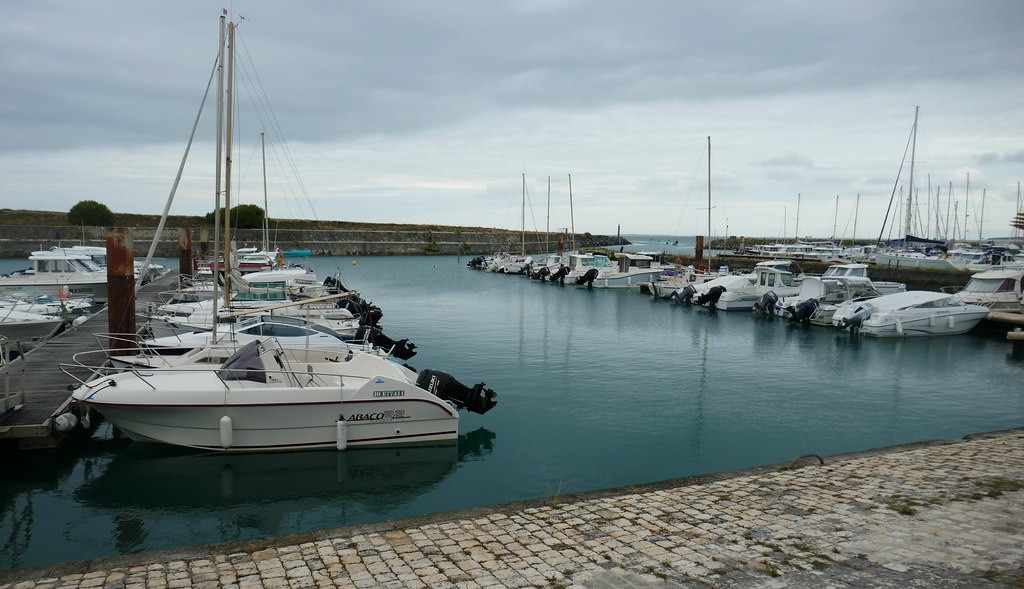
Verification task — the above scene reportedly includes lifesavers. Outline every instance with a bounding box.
[675,258,681,268]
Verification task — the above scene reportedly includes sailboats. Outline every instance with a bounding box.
[56,8,500,456]
[478,101,1024,341]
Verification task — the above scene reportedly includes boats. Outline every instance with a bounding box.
[0,243,175,353]
